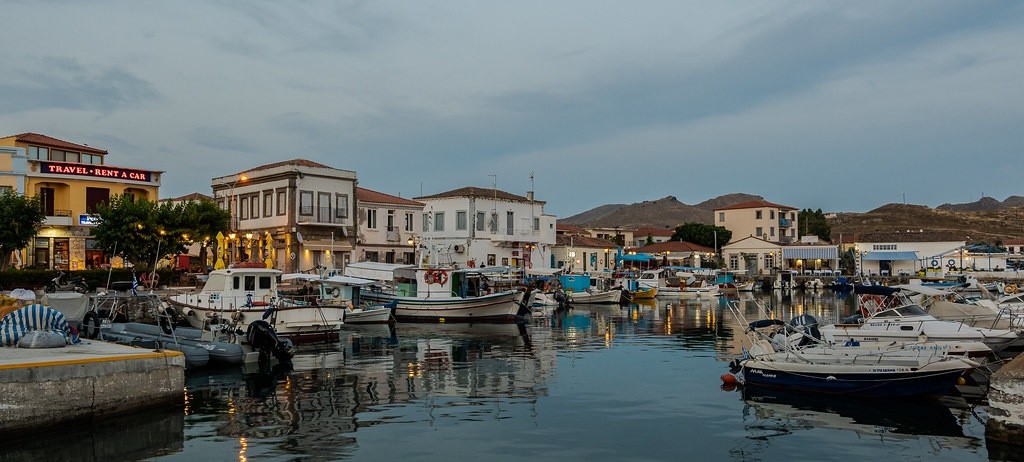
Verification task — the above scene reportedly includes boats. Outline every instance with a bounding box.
[716,266,1024,406]
[35,252,716,373]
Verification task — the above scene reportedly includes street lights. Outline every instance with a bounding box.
[604,246,612,270]
[770,250,781,275]
[228,230,252,262]
[407,234,416,265]
[219,173,248,233]
[856,250,867,276]
[525,242,536,269]
[955,246,969,273]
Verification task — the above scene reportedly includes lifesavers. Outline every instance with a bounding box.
[437,271,448,283]
[859,294,883,317]
[331,287,340,298]
[424,271,436,283]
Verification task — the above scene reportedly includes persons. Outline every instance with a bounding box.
[481,280,492,294]
[139,269,160,290]
[479,261,486,269]
